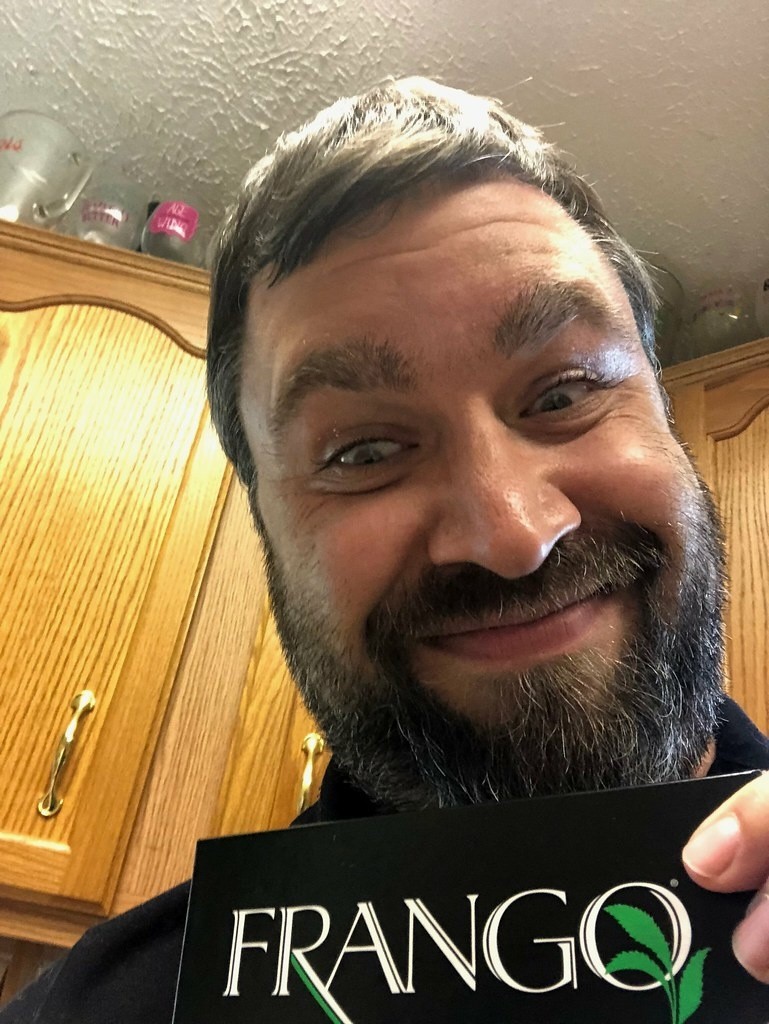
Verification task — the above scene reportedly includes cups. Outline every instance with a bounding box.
[753,276,769,336]
[679,279,757,360]
[0,107,98,232]
[640,259,684,366]
[73,180,153,248]
[139,199,209,264]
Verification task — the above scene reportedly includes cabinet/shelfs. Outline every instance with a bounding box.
[0,221,334,1004]
[659,339,769,738]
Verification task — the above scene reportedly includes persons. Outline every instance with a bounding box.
[0,76,769,1024]
